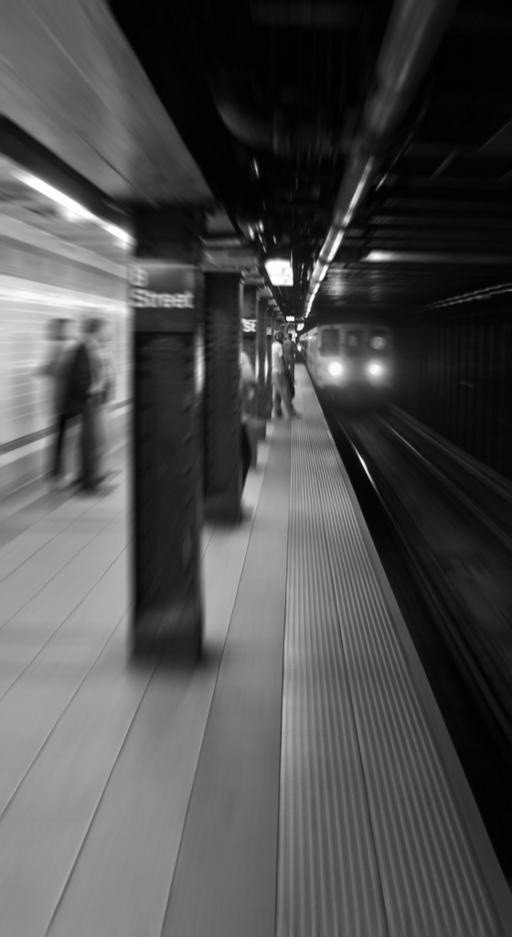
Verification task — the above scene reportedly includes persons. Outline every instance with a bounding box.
[240,326,302,484]
[39,318,114,489]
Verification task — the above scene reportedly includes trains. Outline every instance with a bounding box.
[295,323,399,404]
